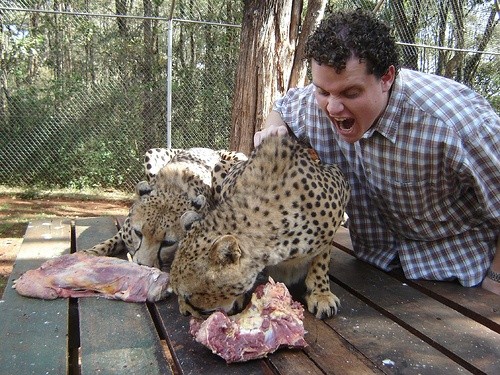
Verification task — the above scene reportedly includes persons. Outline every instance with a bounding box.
[253,7,500,296]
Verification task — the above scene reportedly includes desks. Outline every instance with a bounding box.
[0,215,500,375]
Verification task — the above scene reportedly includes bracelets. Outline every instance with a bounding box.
[486,268,500,283]
